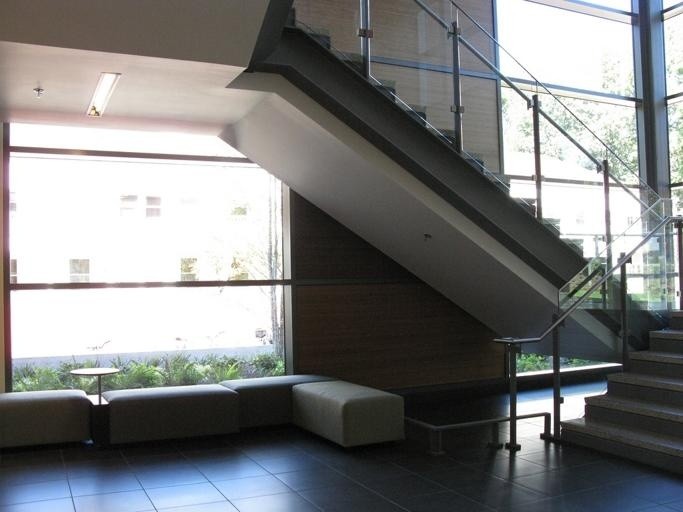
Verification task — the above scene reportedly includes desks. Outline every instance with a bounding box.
[71,368,121,448]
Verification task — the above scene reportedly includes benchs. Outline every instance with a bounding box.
[0,373,406,448]
